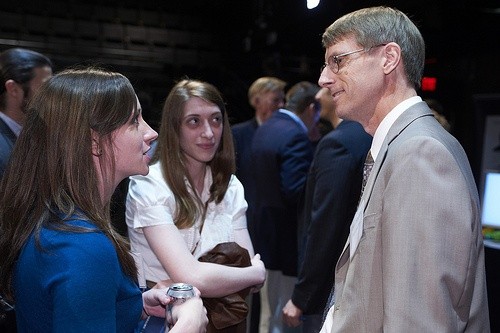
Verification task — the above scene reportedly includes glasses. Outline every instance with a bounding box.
[321,44,387,74]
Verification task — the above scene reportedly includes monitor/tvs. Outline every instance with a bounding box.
[480,169,500,229]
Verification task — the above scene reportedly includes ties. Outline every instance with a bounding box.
[357,150,375,209]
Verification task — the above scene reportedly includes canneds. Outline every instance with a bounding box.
[164,282,195,333]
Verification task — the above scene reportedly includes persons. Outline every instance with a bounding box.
[228,66,383,333]
[0,46,55,175]
[318,6,490,333]
[0,69,160,332]
[124,79,267,333]
